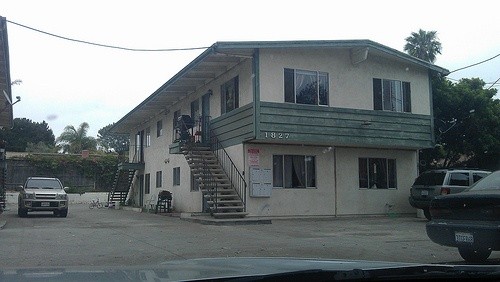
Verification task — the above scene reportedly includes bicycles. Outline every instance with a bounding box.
[88,199,103,209]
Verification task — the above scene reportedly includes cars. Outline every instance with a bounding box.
[424,172,500,262]
[17,177,70,218]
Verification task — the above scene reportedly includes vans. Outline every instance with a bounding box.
[410,170,490,220]
